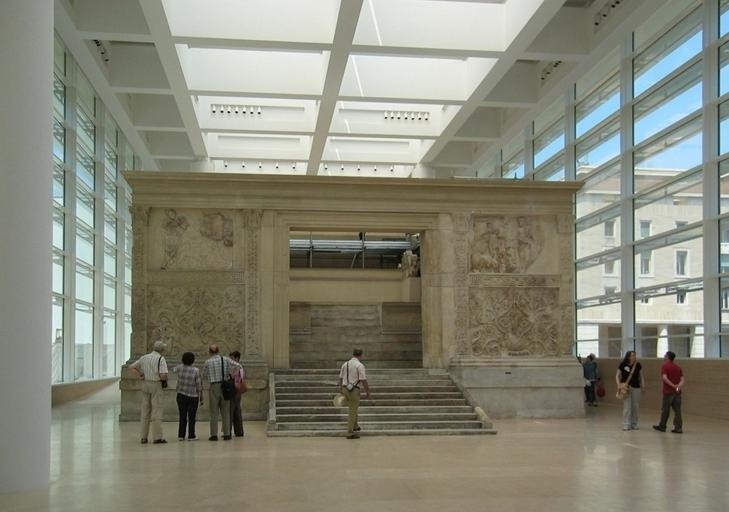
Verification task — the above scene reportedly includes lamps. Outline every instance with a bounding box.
[219,157,302,171]
[382,110,431,123]
[593,0,623,29]
[322,159,400,174]
[538,59,564,83]
[209,103,266,117]
[92,40,111,66]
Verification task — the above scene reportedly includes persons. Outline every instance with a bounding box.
[616,351,645,430]
[175,351,204,441]
[652,352,685,433]
[339,348,369,439]
[582,354,599,406]
[229,351,245,437]
[202,345,242,440]
[131,341,168,444]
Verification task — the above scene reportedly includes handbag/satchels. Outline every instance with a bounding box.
[222,378,234,400]
[616,383,629,401]
[597,383,606,397]
[235,383,248,393]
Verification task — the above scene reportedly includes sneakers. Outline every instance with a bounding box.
[188,436,199,441]
[653,425,665,432]
[209,435,217,441]
[346,434,360,439]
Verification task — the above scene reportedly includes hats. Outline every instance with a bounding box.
[154,341,167,351]
[333,393,347,408]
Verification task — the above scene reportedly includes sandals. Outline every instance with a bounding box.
[153,439,167,444]
